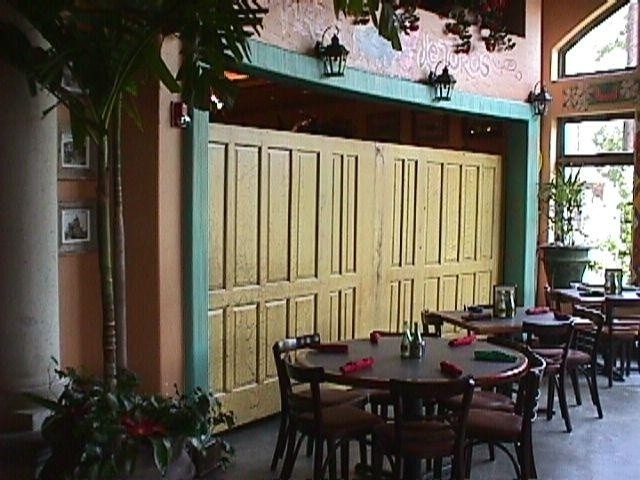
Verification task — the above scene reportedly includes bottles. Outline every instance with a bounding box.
[400,320,423,359]
[496,290,516,318]
[605,273,616,294]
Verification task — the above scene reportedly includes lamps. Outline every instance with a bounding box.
[314,22,348,77]
[430,58,456,101]
[526,79,551,117]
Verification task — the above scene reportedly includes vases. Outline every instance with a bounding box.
[118,440,197,480]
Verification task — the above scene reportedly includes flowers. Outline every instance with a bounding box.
[25,354,237,480]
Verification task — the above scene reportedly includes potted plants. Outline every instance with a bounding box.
[537,164,591,285]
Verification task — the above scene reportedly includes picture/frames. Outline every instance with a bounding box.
[57,120,98,179]
[57,198,98,254]
[604,268,623,295]
